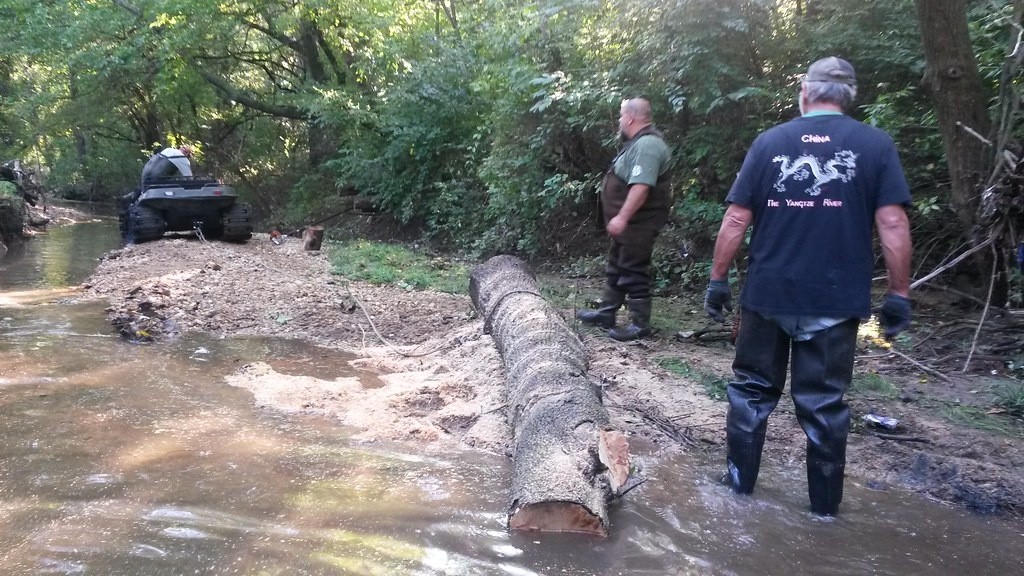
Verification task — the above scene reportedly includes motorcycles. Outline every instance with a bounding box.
[117,174,253,244]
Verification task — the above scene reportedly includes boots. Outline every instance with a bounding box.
[579,284,626,327]
[608,296,652,341]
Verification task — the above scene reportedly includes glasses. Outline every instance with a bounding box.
[623,99,636,112]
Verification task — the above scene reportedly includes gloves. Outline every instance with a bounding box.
[705,278,733,323]
[879,293,911,338]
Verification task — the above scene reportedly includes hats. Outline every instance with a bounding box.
[181,146,192,154]
[805,57,856,85]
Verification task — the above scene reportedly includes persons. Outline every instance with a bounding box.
[577,98,672,342]
[704,56,915,516]
[139,147,193,193]
